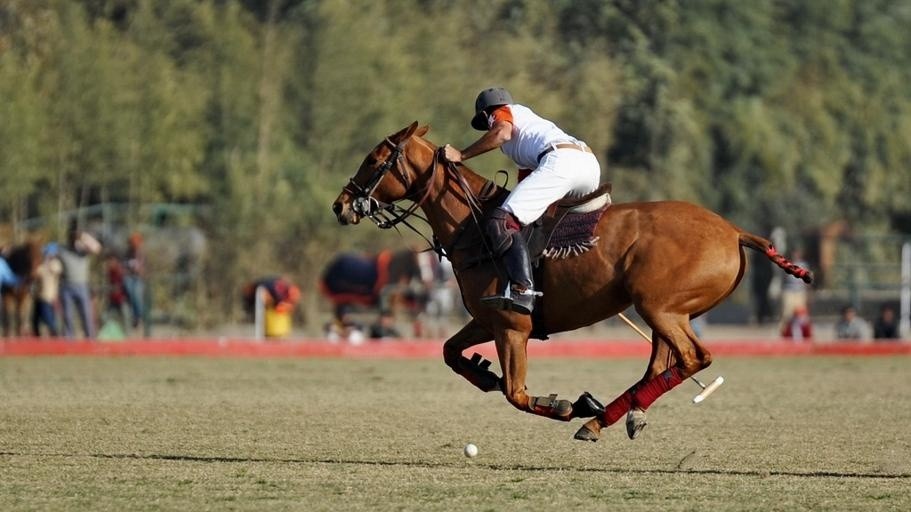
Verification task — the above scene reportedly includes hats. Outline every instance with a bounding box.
[471,88,514,130]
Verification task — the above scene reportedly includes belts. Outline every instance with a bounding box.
[537,143,593,163]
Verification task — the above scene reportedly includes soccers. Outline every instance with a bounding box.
[464,443,478,458]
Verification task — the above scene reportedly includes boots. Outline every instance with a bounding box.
[480,230,536,315]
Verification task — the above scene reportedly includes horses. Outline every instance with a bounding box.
[331,118,816,444]
[322,246,420,330]
[0,237,47,338]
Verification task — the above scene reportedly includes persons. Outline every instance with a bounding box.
[778,241,812,339]
[439,86,601,315]
[873,306,897,339]
[833,303,869,340]
[0,213,151,341]
